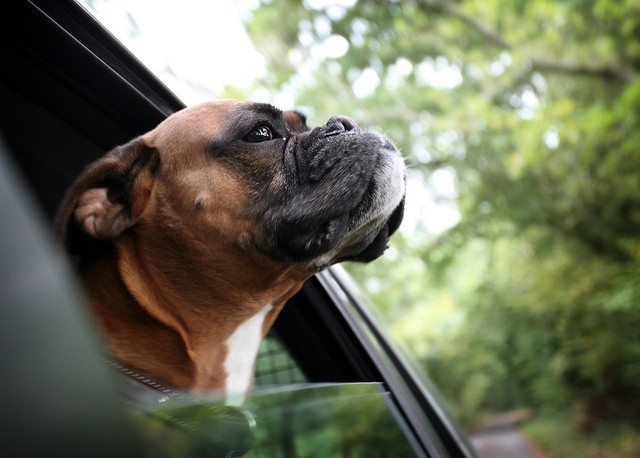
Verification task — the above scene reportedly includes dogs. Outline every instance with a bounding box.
[53,96,413,409]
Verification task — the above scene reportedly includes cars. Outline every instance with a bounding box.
[1,0,474,454]
